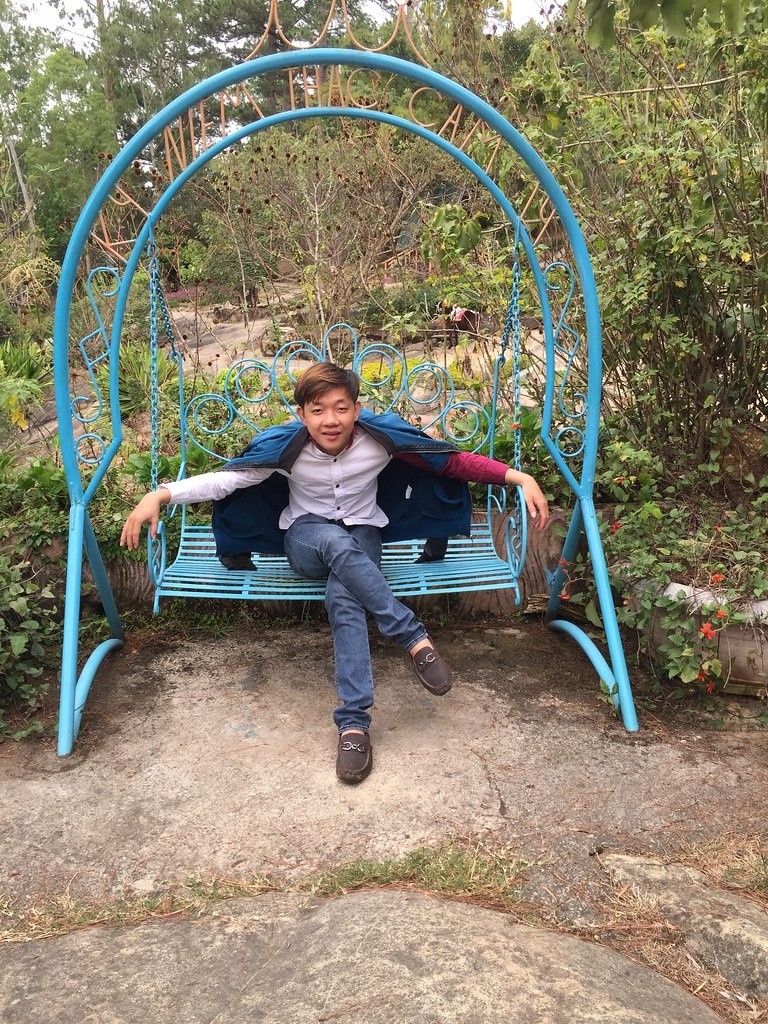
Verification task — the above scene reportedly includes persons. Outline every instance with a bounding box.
[119,362,550,784]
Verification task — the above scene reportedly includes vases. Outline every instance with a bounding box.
[608,560,768,697]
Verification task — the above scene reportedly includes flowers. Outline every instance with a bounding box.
[557,505,768,700]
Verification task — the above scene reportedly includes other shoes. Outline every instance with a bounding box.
[336,729,374,786]
[410,635,455,696]
